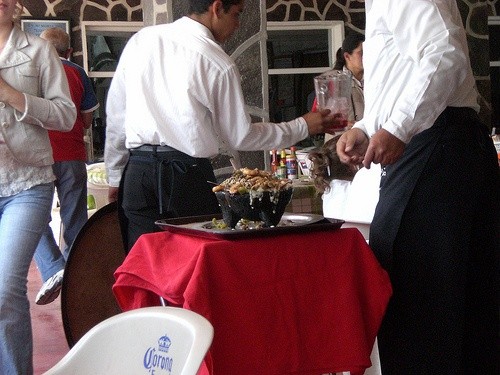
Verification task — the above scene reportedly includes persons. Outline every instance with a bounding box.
[337,0,500,375]
[0,0,77,375]
[310,32,365,181]
[103,0,349,256]
[33,27,100,305]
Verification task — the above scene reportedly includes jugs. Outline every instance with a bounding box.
[314,69,352,133]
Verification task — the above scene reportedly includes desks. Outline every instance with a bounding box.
[112,229,393,375]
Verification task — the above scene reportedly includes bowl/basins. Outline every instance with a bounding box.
[214,187,291,228]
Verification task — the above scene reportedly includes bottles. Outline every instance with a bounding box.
[290,146,297,157]
[278,149,287,179]
[271,149,279,173]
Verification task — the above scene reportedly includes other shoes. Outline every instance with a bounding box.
[35,269,65,305]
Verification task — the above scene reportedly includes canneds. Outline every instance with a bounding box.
[276,155,297,180]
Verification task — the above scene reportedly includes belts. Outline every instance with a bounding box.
[128,145,176,153]
